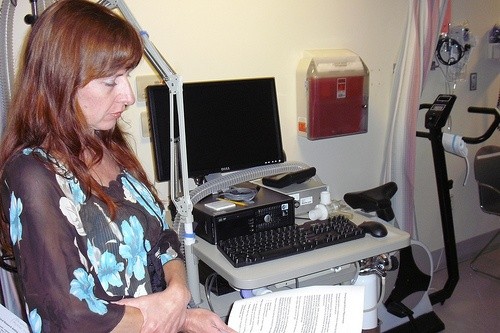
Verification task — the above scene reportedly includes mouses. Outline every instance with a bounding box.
[358,221,388,238]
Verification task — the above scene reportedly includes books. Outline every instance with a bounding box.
[227,284,366,333]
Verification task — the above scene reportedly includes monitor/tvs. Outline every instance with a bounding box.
[145,77,285,196]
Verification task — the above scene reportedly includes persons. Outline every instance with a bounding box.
[0,0,239,333]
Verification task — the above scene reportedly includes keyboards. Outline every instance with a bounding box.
[217,214,365,267]
